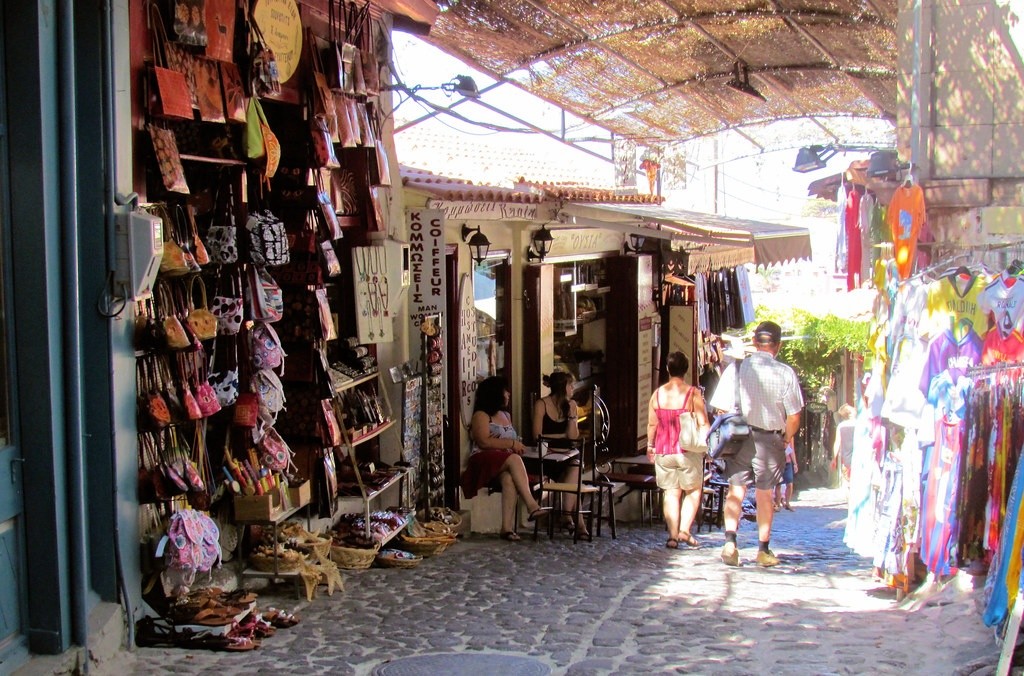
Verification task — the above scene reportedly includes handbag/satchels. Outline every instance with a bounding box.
[679,387,708,453]
[705,413,750,460]
[133,0,393,580]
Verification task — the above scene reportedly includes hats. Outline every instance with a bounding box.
[755,321,781,343]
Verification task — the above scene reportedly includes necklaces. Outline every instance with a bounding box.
[356,246,388,340]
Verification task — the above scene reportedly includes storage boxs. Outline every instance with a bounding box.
[234,479,311,521]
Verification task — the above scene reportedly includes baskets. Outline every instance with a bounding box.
[251,507,462,577]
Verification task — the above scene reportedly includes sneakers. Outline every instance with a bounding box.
[757,550,780,566]
[722,542,740,566]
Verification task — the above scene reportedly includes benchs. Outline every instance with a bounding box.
[597,473,658,527]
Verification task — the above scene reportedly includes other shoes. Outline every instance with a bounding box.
[775,504,781,511]
[135,587,300,651]
[785,504,795,512]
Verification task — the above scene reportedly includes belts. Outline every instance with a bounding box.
[747,425,782,435]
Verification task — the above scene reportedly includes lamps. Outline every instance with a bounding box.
[528,224,555,262]
[462,224,492,265]
[792,144,881,173]
[724,61,767,102]
[624,224,645,254]
[380,75,481,99]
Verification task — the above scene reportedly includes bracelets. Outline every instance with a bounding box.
[569,416,577,419]
[512,440,514,448]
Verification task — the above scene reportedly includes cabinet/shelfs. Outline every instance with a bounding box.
[236,501,332,599]
[572,373,604,472]
[332,372,416,547]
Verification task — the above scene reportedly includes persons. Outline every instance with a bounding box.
[709,321,794,567]
[473,375,548,541]
[773,445,797,510]
[833,403,857,491]
[532,372,593,540]
[647,352,710,548]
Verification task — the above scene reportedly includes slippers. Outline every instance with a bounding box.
[580,525,588,537]
[500,531,521,541]
[562,516,576,531]
[526,508,548,522]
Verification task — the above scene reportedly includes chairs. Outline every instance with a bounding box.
[534,434,599,544]
[697,457,715,532]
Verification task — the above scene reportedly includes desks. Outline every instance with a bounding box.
[519,445,581,466]
[614,455,656,465]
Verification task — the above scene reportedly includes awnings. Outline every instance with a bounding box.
[556,201,811,273]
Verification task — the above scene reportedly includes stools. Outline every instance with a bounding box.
[461,487,528,532]
[703,481,730,528]
[582,480,616,540]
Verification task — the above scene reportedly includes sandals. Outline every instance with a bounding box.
[666,538,679,549]
[677,530,700,546]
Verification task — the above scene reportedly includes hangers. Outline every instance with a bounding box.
[969,359,1024,406]
[1003,311,1012,337]
[839,160,914,209]
[916,256,1024,282]
